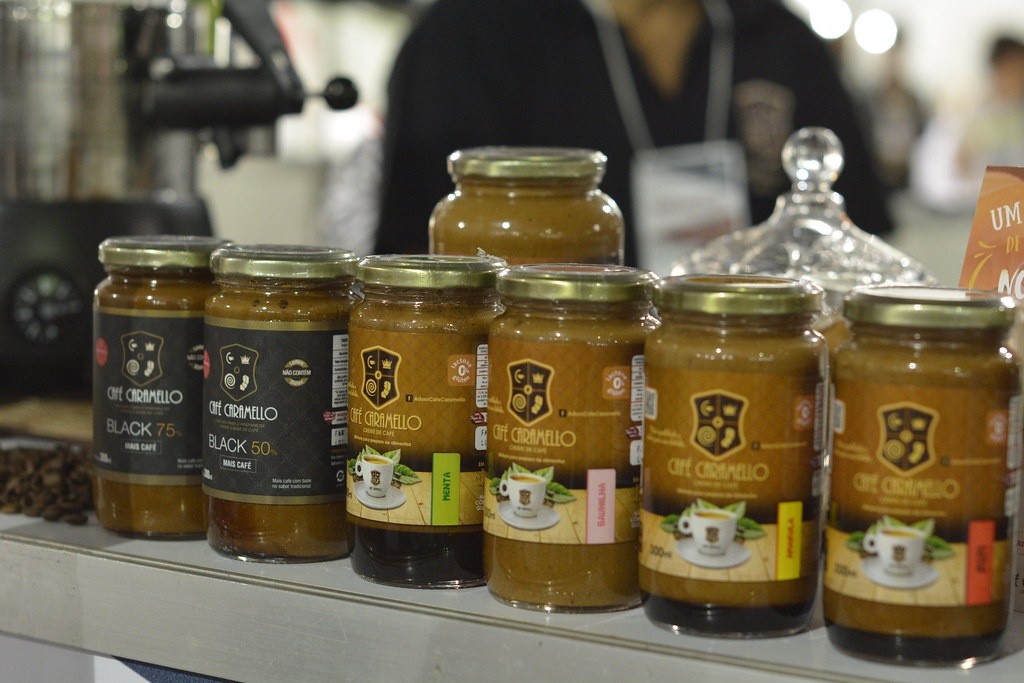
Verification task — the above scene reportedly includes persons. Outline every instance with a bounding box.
[370,1,898,279]
